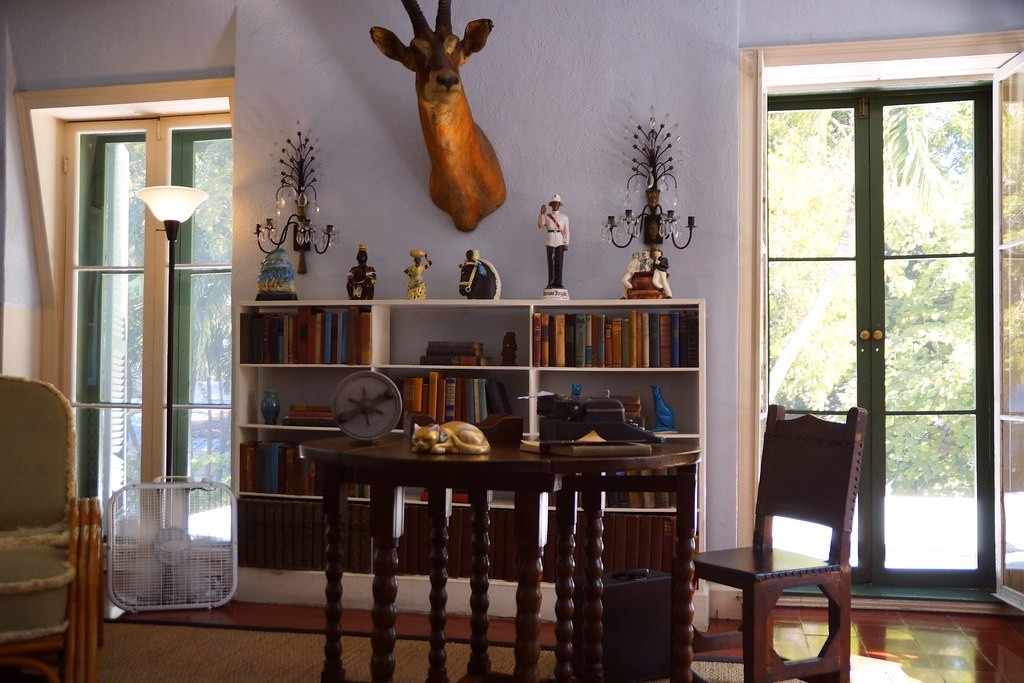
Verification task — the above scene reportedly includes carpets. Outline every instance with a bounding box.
[16,619,792,683]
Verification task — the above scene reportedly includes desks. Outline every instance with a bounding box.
[299,434,702,683]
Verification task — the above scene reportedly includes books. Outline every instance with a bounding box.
[532,310,699,368]
[419,489,468,504]
[240,439,371,498]
[401,370,513,441]
[547,466,678,508]
[591,394,645,430]
[282,405,340,427]
[236,494,678,584]
[419,340,493,366]
[240,305,372,365]
[519,431,652,457]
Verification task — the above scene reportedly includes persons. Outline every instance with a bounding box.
[621,249,672,299]
[346,244,376,300]
[404,251,432,301]
[538,194,570,289]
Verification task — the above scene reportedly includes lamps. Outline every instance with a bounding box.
[251,119,339,275]
[135,185,210,482]
[601,107,699,251]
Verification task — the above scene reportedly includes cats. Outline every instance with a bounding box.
[411,421,492,456]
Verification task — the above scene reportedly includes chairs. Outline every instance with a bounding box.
[690,403,869,683]
[0,374,104,683]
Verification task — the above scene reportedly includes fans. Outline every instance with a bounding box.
[106,475,237,614]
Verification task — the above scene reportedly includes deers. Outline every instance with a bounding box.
[368,0,509,233]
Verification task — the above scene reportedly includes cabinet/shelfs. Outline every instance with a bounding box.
[235,299,710,632]
